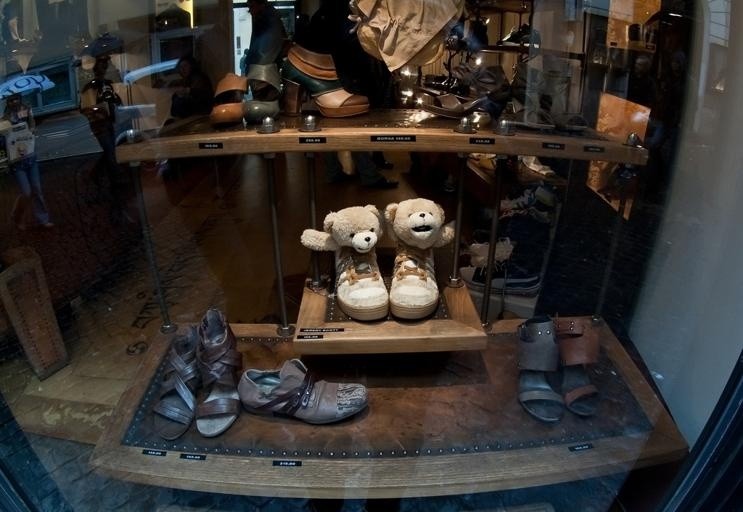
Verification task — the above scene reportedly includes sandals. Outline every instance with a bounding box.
[153,308,241,440]
[516,310,601,423]
[209,62,282,124]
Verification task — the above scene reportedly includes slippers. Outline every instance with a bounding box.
[421,94,590,133]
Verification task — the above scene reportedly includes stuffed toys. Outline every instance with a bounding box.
[384,198,453,251]
[299,204,384,253]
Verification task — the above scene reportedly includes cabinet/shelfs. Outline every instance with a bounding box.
[86,115,689,502]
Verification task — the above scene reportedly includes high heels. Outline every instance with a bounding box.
[281,43,370,120]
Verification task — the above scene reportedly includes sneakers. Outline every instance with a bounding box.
[32,218,56,229]
[458,191,558,296]
[9,216,26,231]
[335,244,390,322]
[238,359,368,425]
[389,239,439,320]
[498,23,543,50]
[109,209,137,224]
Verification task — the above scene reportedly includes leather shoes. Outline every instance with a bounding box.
[441,169,457,193]
[325,171,399,190]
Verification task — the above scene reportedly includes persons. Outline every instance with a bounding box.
[349,150,400,188]
[0,94,57,236]
